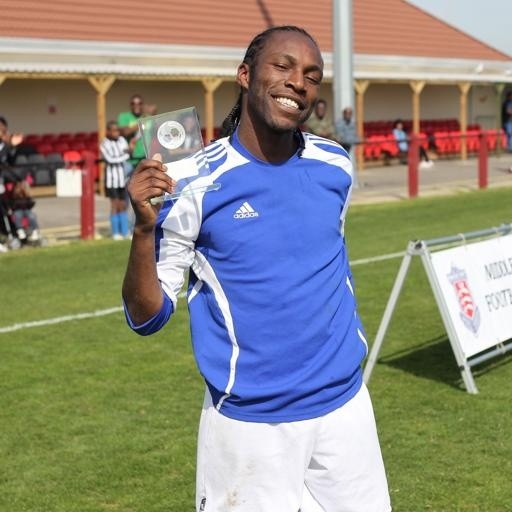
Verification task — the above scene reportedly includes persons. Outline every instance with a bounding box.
[501,90,512,154]
[121,25,392,512]
[394,118,433,169]
[160,113,201,166]
[0,116,42,254]
[333,105,366,154]
[305,96,337,143]
[116,93,156,242]
[97,119,135,242]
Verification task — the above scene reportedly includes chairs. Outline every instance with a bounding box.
[10,127,223,187]
[362,118,509,160]
